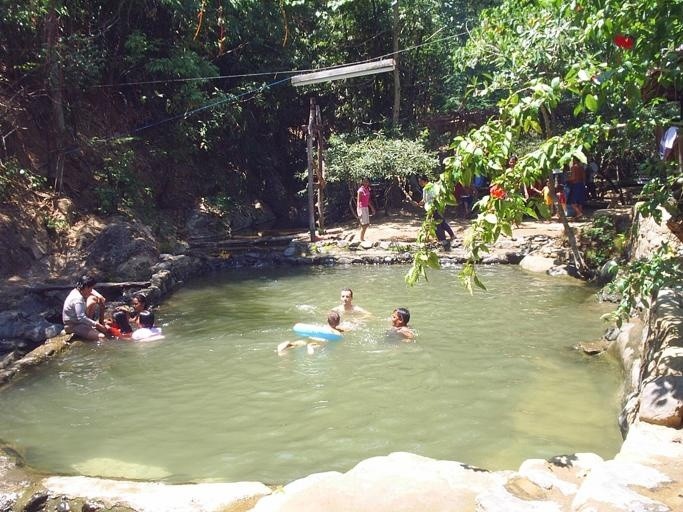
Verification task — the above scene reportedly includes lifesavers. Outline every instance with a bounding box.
[292,322,343,341]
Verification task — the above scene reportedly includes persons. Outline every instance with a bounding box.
[102,310,132,342]
[416,174,457,242]
[61,274,110,343]
[330,287,372,322]
[356,176,375,241]
[114,293,148,328]
[455,154,601,220]
[131,309,164,341]
[390,307,414,341]
[276,310,344,354]
